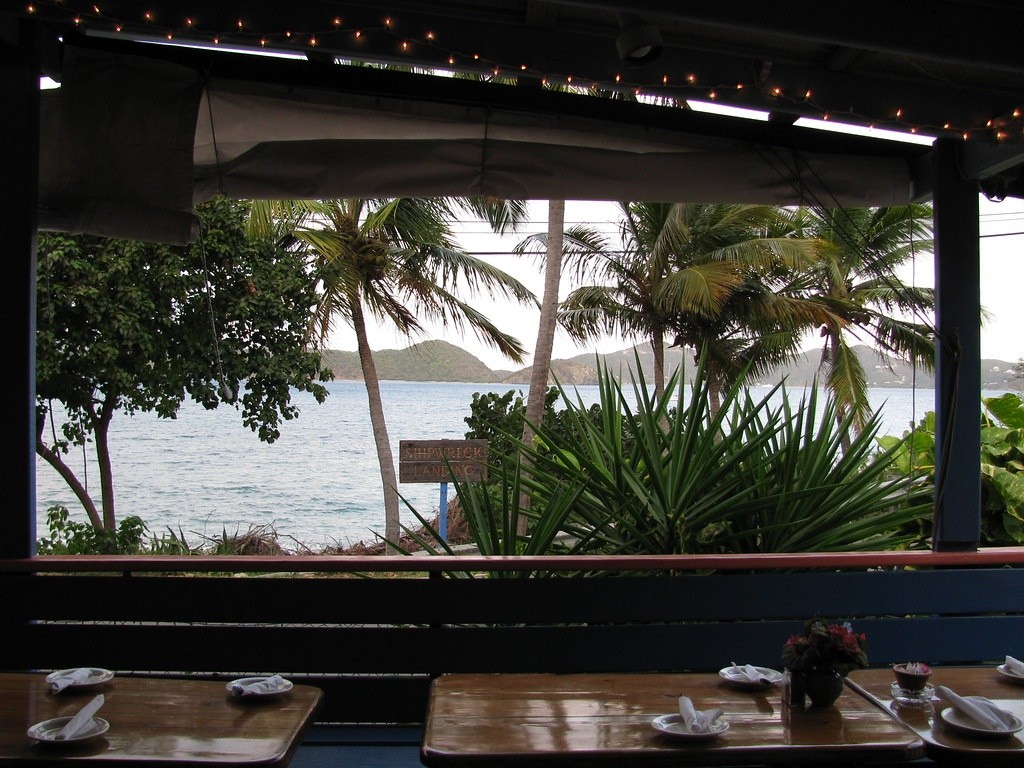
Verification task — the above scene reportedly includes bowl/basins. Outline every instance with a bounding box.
[890,680,935,709]
[892,662,932,693]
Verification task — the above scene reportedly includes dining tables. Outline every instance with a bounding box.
[844,667,1024,768]
[0,672,326,768]
[421,671,925,768]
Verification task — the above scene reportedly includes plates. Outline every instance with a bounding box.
[47,668,114,686]
[651,713,731,738]
[997,664,1024,679]
[225,676,293,694]
[27,716,109,741]
[720,666,782,686]
[940,706,1022,734]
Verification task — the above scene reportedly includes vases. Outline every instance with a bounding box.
[805,667,841,707]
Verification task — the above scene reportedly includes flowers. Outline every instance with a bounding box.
[781,620,869,675]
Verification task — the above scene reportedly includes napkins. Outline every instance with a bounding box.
[56,692,105,741]
[731,661,781,689]
[677,695,724,734]
[1005,655,1024,678]
[230,673,282,698]
[51,668,90,693]
[936,681,1009,733]
